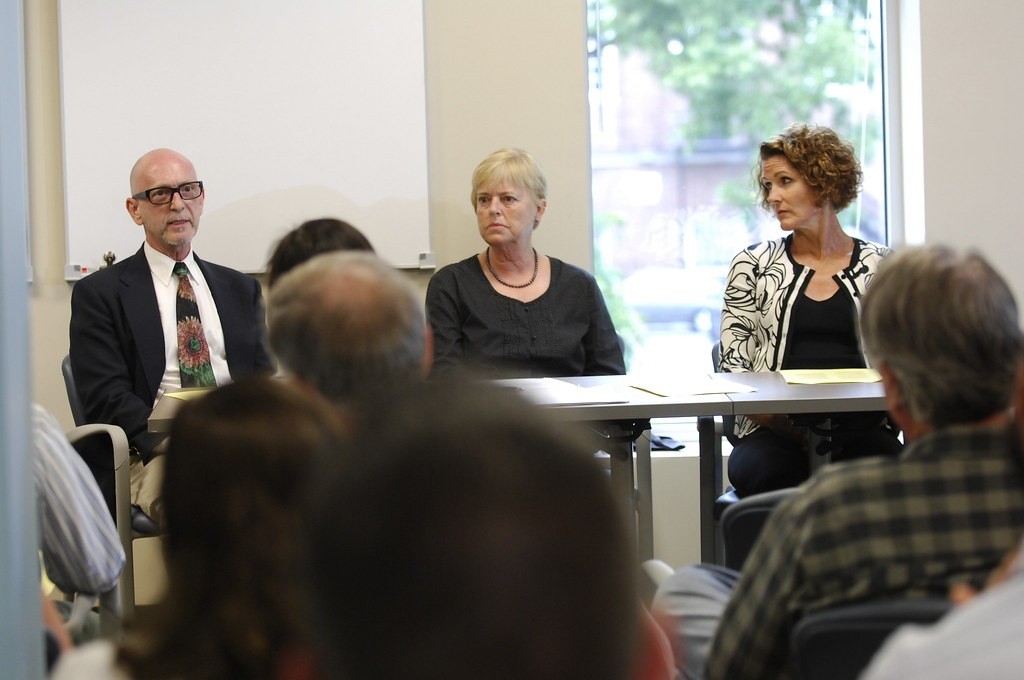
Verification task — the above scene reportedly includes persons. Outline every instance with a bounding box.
[31,400,126,599]
[68,148,278,524]
[424,148,628,377]
[650,246,1022,679]
[51,378,677,680]
[717,122,902,498]
[268,218,376,287]
[265,250,435,417]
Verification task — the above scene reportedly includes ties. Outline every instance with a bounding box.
[173,262,218,388]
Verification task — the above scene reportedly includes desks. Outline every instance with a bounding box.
[710,367,890,565]
[490,374,732,564]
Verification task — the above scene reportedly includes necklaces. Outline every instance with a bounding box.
[486,245,538,288]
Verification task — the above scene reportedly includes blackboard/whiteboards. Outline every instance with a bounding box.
[60,0,437,283]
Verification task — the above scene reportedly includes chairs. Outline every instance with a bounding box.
[61,352,160,621]
[593,334,946,680]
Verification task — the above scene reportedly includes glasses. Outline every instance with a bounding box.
[133,181,203,205]
